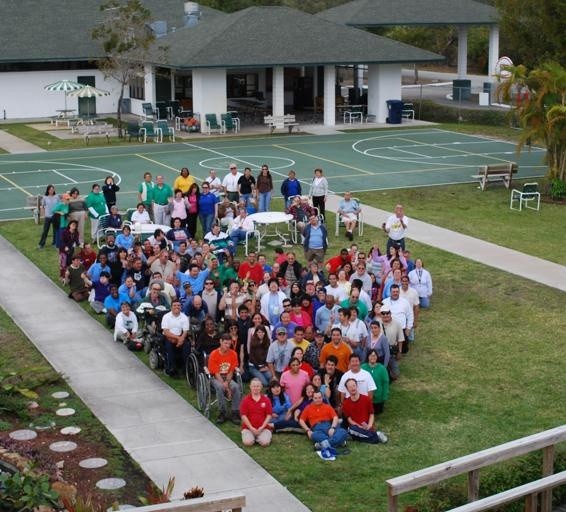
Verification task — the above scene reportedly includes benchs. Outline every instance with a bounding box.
[79,125,117,145]
[470,163,519,192]
[23,195,45,224]
[264,114,299,135]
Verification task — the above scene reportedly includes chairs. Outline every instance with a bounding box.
[48,108,85,134]
[335,198,363,237]
[510,182,540,213]
[125,103,241,143]
[125,208,153,224]
[97,214,131,249]
[401,103,415,121]
[287,194,329,245]
[214,202,239,233]
[226,223,260,257]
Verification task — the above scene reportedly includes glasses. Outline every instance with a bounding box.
[381,312,389,314]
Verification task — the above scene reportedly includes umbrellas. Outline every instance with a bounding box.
[42,80,85,110]
[66,83,110,118]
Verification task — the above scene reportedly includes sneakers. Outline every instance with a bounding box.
[376,431,387,443]
[217,414,240,424]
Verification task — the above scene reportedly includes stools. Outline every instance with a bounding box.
[344,110,363,124]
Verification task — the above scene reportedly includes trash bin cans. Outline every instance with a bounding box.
[119,97,131,115]
[385,99,404,124]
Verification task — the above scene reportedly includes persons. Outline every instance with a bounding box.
[131,203,150,242]
[281,170,302,208]
[286,196,315,232]
[385,205,408,255]
[309,170,328,223]
[106,205,123,229]
[38,176,120,248]
[337,192,361,241]
[292,76,305,111]
[138,164,273,254]
[302,215,327,270]
[58,219,235,374]
[184,243,433,451]
[184,114,197,131]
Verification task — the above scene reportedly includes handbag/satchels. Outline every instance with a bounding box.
[389,344,398,354]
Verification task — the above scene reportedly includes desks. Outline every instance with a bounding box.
[128,225,172,239]
[249,211,293,247]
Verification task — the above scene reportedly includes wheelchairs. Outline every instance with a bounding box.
[183,320,221,393]
[143,292,170,354]
[197,343,244,420]
[148,310,194,375]
